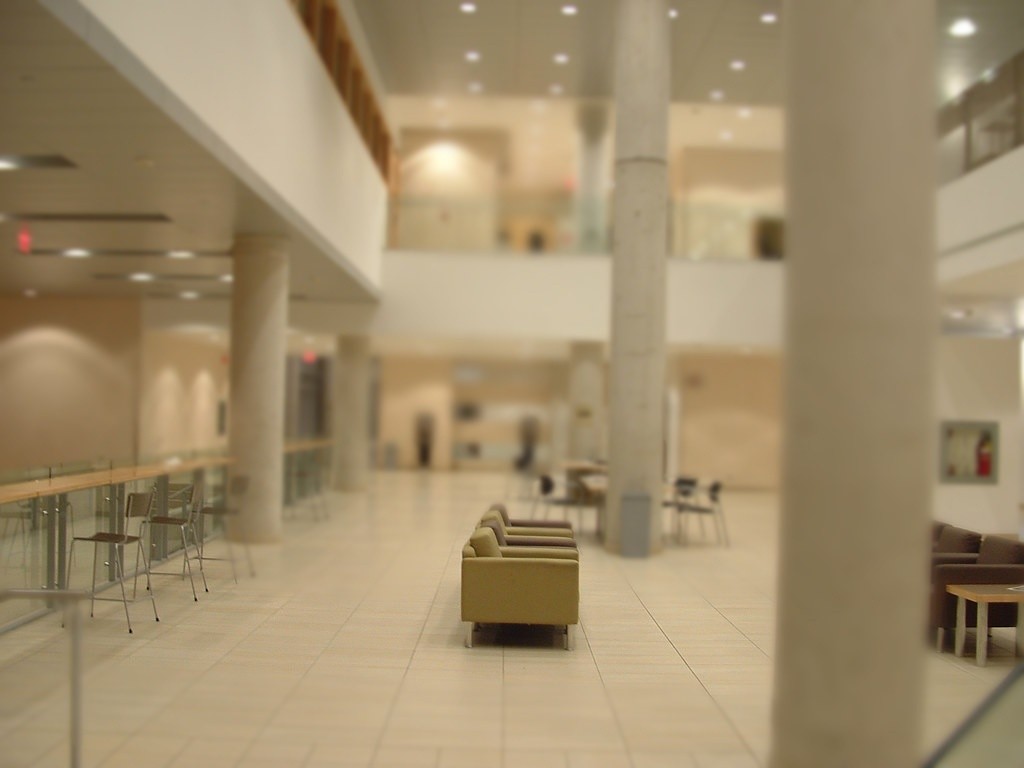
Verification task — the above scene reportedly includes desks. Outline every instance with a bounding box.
[581,475,608,537]
[0,455,230,610]
[557,458,606,502]
[945,584,1024,667]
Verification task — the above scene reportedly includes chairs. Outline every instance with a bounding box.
[133,479,209,600]
[662,475,729,545]
[928,519,1024,653]
[527,474,577,520]
[461,501,580,651]
[60,488,160,634]
[200,474,255,584]
